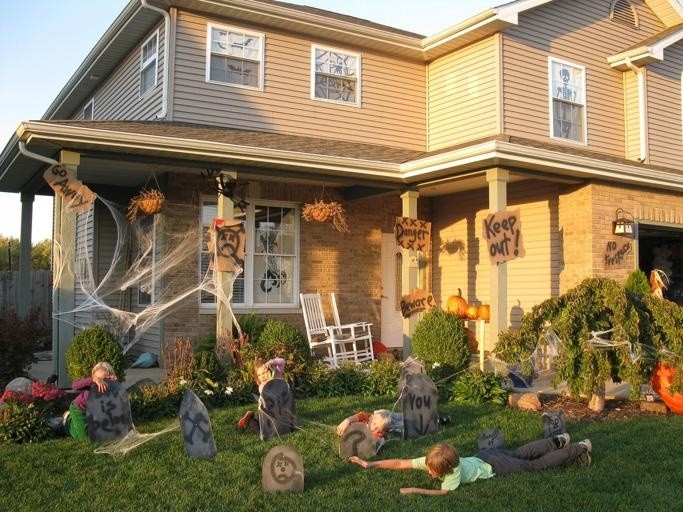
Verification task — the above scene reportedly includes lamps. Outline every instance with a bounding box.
[611,207,637,236]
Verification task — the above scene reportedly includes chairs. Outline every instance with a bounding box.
[296,291,377,377]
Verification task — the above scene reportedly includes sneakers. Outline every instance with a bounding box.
[576,438,593,467]
[552,433,571,449]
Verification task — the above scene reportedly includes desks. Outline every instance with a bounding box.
[461,318,491,372]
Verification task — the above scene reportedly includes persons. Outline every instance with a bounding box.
[57,362,117,441]
[336,409,392,438]
[348,432,592,495]
[238,357,285,432]
